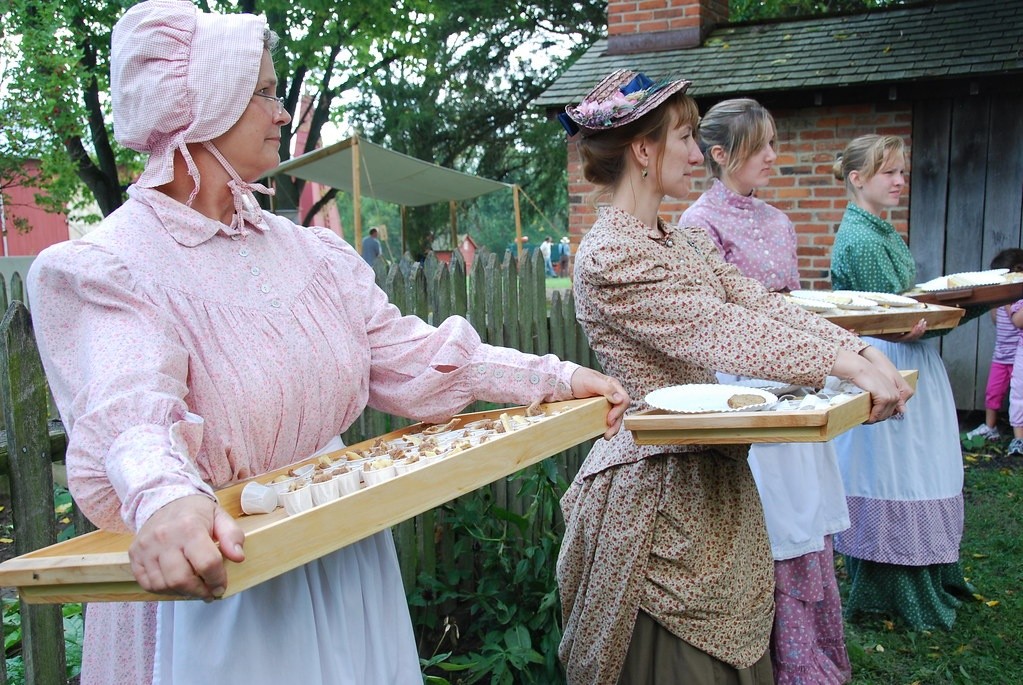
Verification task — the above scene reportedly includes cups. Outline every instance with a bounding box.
[240,411,553,516]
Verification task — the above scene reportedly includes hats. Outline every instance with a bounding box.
[558,69,692,139]
[110,0,276,238]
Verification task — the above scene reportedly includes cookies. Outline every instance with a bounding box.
[727,394,766,410]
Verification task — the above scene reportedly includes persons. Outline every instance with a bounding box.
[556,68,915,685]
[822,134,979,635]
[965,249,1023,455]
[677,99,928,685]
[540,236,559,278]
[362,228,382,266]
[26,0,631,685]
[558,236,570,278]
[419,231,434,267]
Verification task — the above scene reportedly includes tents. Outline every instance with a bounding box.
[262,135,524,269]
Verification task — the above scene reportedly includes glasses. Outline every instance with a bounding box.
[253,93,284,115]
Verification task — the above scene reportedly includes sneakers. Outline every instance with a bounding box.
[966,423,1001,441]
[1008,438,1023,456]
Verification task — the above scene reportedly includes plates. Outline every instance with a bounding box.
[916,269,1012,292]
[646,384,778,413]
[730,379,800,396]
[788,289,914,315]
